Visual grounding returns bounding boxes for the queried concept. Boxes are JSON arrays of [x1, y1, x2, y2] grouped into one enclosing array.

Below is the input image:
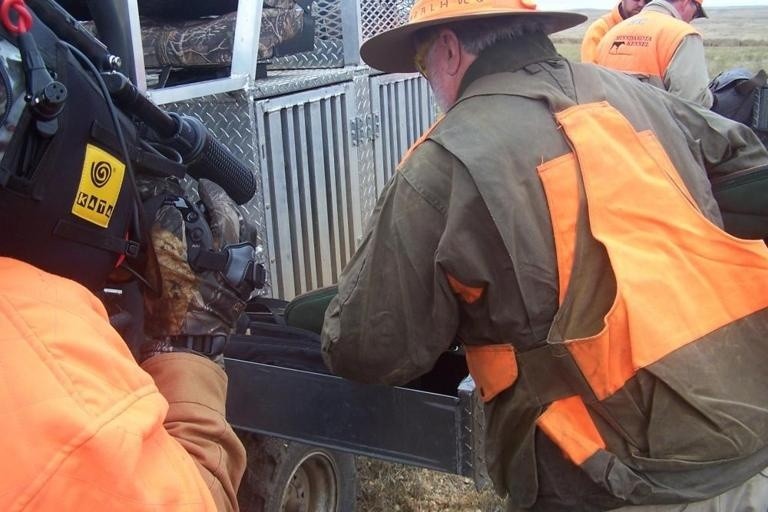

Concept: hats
[[360, 0, 588, 73], [694, 0, 707, 17]]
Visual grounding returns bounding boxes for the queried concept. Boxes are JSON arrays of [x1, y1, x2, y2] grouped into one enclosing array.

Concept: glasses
[[694, 3, 704, 18], [413, 26, 440, 78]]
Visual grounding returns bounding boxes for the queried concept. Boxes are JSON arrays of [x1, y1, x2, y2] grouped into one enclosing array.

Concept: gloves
[[140, 178, 257, 370]]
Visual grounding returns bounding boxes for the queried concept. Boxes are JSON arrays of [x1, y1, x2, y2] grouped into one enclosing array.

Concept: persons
[[575, 0, 654, 68], [313, 0, 768, 509], [604, 0, 722, 113], [1, 172, 262, 512]]
